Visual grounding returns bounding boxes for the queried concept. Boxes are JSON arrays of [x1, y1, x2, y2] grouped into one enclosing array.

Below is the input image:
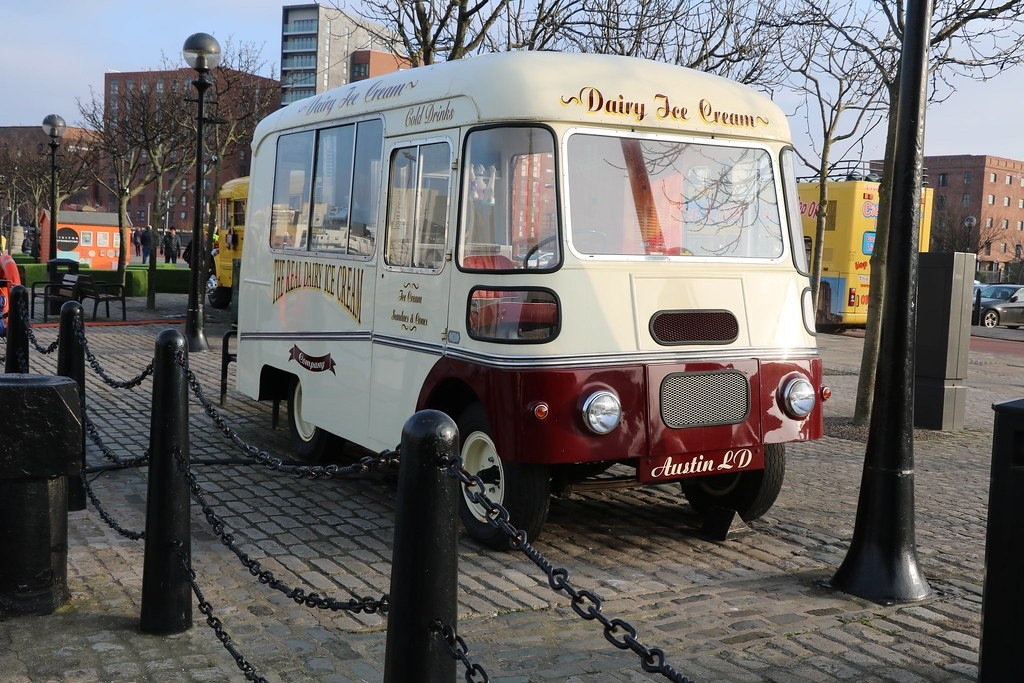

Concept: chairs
[[464, 255, 514, 270]]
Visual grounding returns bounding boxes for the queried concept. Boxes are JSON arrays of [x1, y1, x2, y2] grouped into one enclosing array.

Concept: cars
[[971, 285, 1024, 329]]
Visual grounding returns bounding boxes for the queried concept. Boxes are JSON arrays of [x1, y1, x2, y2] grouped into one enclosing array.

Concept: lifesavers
[[0, 254, 21, 304]]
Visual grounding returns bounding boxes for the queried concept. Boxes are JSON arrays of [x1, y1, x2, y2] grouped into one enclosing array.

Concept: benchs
[[30, 272, 127, 322]]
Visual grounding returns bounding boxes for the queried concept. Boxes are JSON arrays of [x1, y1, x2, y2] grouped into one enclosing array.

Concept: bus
[[795, 160, 935, 334], [203, 171, 326, 309], [236, 50, 832, 554]]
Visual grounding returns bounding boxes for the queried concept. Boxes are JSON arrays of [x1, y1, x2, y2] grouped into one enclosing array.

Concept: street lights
[[43, 114, 66, 260], [183, 33, 222, 352], [965, 216, 976, 253]]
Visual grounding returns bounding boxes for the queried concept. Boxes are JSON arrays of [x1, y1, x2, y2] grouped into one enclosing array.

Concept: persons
[[22, 235, 41, 257], [160, 226, 181, 264], [133, 225, 152, 264], [182, 230, 219, 270]]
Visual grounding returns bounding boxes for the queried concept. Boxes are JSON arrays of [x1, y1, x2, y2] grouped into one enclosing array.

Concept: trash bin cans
[[0, 373, 87, 624], [47, 259, 79, 315]]
